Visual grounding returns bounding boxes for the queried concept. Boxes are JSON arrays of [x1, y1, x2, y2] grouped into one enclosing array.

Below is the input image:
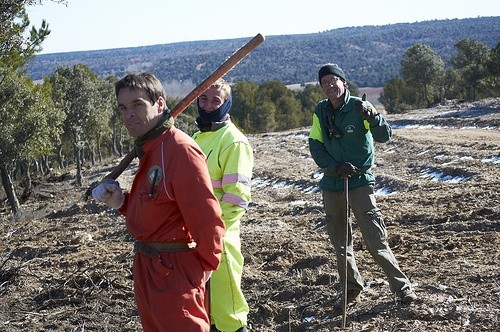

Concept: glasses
[[320, 77, 339, 86]]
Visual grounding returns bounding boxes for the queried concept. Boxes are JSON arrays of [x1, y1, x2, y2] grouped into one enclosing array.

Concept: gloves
[[336, 161, 358, 178], [91, 178, 125, 209], [357, 93, 378, 121]]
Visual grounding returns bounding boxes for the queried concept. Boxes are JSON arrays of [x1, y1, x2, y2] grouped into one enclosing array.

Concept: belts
[[133, 241, 188, 253]]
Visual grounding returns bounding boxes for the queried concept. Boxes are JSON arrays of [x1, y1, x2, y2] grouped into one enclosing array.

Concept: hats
[[319, 63, 346, 88]]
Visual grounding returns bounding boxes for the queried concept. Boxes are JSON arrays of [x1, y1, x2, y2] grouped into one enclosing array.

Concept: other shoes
[[347, 289, 360, 303], [401, 291, 417, 303]]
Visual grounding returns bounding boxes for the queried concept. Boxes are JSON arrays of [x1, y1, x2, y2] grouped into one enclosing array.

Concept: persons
[[191, 77, 254, 332], [92, 71, 225, 332], [309, 63, 417, 307]]
